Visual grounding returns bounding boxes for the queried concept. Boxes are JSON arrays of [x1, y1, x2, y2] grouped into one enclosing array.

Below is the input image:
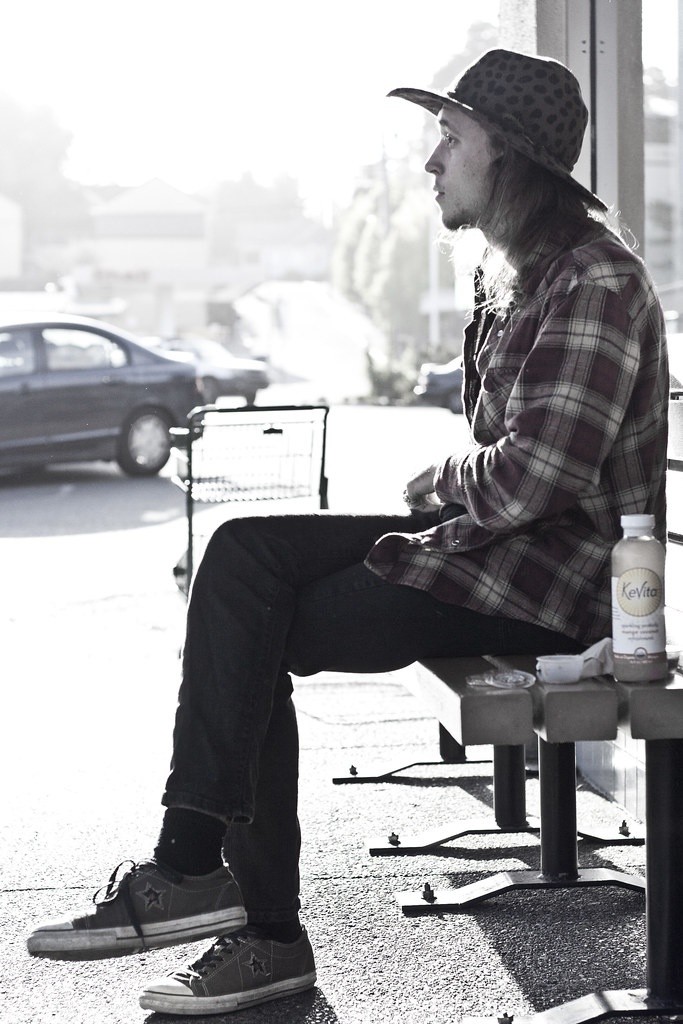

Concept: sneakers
[[140, 926, 316, 1013], [26, 863, 244, 959]]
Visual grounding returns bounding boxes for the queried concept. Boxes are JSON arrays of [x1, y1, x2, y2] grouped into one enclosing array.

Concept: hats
[[386, 49, 611, 214]]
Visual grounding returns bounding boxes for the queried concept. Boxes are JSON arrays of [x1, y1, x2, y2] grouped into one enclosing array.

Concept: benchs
[[407, 652, 683, 1024]]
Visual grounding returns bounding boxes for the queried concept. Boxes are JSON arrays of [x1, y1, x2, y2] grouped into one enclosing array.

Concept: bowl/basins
[[535, 656, 583, 684]]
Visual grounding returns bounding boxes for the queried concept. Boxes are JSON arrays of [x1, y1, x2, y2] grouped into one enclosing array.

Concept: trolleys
[[168, 401, 331, 660]]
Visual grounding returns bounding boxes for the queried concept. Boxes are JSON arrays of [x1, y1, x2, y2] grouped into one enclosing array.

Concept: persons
[[29, 49, 670, 1013]]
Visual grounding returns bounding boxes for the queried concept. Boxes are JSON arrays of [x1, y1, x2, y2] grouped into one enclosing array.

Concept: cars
[[0, 305, 207, 478], [155, 333, 271, 406], [412, 353, 464, 418]]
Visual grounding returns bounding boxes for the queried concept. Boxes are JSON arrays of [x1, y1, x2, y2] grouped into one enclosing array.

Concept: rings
[[403, 490, 418, 503]]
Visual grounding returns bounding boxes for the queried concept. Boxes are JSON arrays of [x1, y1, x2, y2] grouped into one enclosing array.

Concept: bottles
[[609, 514, 671, 683]]
